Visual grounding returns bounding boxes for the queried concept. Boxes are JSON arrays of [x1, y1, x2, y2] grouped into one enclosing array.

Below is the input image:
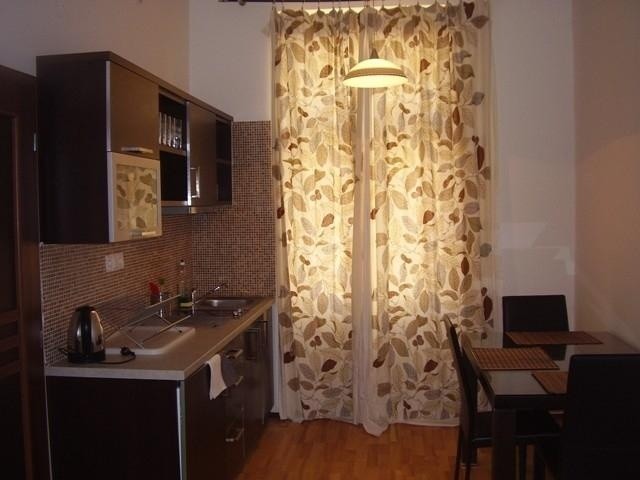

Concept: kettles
[[67, 305, 106, 365]]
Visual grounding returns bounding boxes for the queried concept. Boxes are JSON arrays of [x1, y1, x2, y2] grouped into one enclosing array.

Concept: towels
[[204, 352, 229, 402]]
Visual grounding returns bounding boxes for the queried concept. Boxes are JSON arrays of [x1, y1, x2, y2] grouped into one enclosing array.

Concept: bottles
[[177, 259, 193, 307]]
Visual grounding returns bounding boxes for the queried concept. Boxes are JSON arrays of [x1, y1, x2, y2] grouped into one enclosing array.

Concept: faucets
[[192, 282, 228, 304]]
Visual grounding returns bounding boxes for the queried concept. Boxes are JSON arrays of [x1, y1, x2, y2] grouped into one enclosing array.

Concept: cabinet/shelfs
[[186, 351, 246, 480], [159, 92, 192, 206], [239, 307, 274, 457], [106, 63, 163, 242], [191, 102, 234, 207]]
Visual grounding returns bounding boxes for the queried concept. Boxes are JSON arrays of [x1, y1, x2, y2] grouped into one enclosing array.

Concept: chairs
[[440, 290, 639, 479]]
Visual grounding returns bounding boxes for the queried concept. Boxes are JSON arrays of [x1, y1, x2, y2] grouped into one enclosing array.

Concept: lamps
[[340, 4, 409, 88]]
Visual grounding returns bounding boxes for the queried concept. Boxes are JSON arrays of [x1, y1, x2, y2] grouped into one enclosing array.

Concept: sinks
[[192, 297, 256, 311]]
